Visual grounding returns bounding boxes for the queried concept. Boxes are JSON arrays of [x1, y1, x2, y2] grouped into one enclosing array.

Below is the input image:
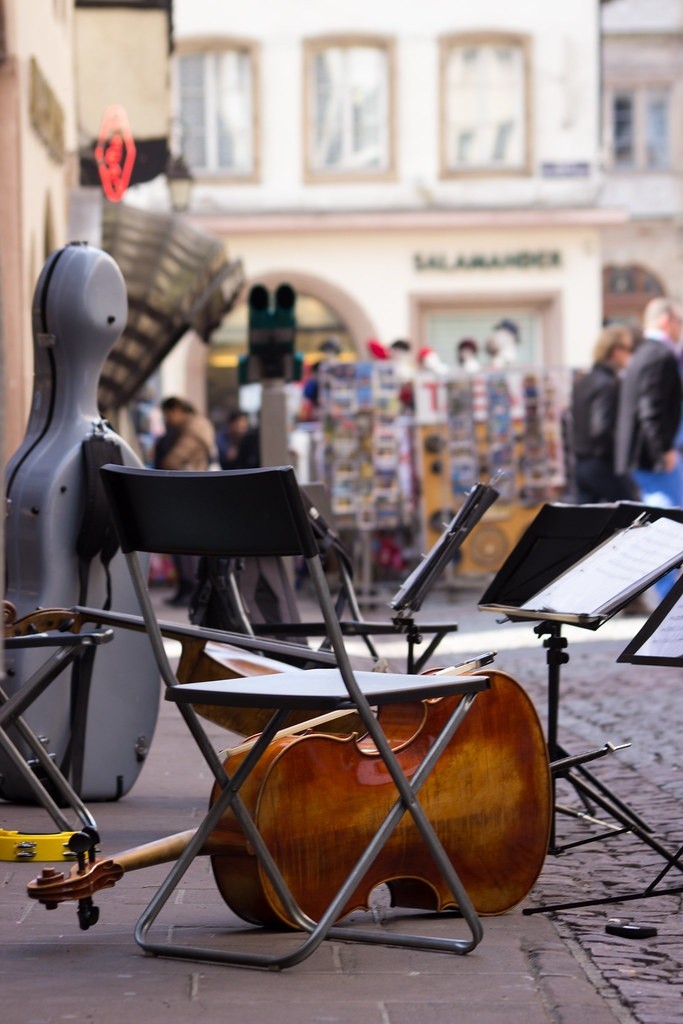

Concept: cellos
[[0, 598, 389, 739], [28, 670, 555, 932]]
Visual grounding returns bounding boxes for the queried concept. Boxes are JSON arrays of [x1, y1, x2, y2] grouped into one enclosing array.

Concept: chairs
[[100, 461, 491, 977]]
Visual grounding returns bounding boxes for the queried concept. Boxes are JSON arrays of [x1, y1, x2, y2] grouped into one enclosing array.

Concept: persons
[[559, 297, 683, 610], [150, 398, 260, 606]]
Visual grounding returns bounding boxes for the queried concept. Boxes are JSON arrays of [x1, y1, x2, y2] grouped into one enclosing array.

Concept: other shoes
[[165, 591, 197, 607]]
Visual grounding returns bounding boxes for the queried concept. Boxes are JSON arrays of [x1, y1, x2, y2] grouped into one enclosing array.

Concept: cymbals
[[0, 827, 101, 862]]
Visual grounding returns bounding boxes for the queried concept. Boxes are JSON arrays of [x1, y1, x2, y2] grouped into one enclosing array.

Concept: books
[[477, 500, 683, 622]]
